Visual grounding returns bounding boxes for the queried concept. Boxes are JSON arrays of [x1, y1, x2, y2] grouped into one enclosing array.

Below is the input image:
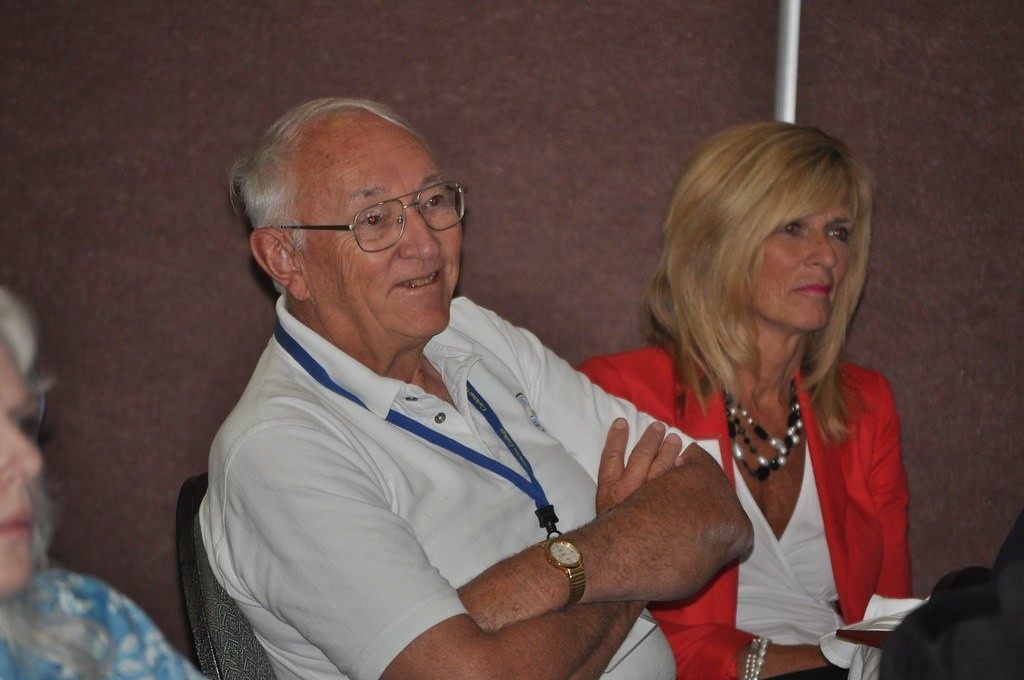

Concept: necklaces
[[723, 380, 804, 483]]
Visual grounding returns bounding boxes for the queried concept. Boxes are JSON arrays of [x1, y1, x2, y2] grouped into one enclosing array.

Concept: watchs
[[537, 537, 584, 608]]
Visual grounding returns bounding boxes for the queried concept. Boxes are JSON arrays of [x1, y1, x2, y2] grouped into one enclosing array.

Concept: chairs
[[174, 470, 276, 680]]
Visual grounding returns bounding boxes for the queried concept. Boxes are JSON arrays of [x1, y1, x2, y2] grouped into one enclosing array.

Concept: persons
[[197, 99, 759, 680], [0, 290, 212, 679], [577, 119, 912, 680]]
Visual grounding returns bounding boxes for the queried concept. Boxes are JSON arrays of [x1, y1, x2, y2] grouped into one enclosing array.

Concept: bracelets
[[744, 635, 770, 680]]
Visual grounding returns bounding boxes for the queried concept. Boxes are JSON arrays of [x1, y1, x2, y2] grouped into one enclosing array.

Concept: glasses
[[278, 180, 467, 253]]
